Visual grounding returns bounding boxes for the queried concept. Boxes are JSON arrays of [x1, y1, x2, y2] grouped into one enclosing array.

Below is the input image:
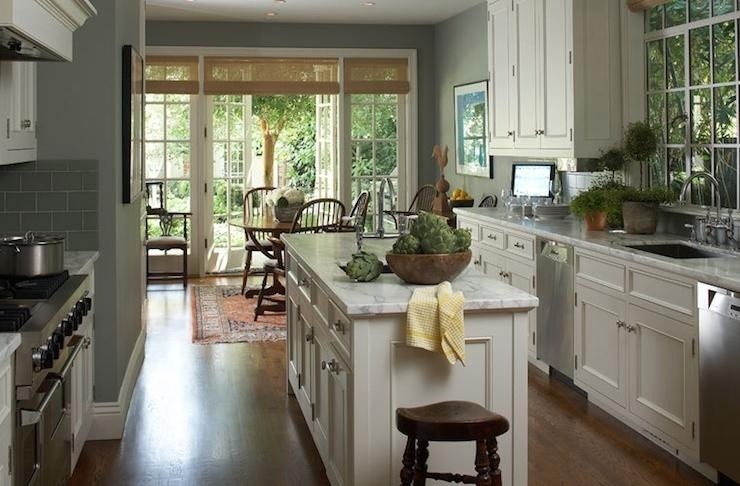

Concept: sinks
[[621, 243, 728, 258]]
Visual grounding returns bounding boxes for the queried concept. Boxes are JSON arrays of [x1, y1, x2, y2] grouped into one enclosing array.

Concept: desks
[[227, 213, 339, 315]]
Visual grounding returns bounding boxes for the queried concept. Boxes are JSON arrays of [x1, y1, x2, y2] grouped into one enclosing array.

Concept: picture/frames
[[123, 44, 145, 204], [453, 79, 493, 178]]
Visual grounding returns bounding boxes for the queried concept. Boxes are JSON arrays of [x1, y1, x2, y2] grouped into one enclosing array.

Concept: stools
[[396, 400, 509, 486]]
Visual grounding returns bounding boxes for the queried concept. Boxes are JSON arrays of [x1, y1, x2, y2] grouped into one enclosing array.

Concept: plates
[[534, 204, 572, 221]]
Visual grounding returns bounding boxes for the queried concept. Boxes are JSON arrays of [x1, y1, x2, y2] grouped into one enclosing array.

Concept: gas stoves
[[0, 271, 91, 373]]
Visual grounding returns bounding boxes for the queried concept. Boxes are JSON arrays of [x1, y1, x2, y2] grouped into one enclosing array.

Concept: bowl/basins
[[448, 199, 474, 212], [274, 203, 302, 224], [384, 252, 473, 286]]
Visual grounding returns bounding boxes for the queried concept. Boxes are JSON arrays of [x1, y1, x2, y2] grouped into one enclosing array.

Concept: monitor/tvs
[[511, 163, 555, 197]]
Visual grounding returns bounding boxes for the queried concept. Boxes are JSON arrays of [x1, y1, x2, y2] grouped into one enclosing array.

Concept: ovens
[[11, 332, 86, 485]]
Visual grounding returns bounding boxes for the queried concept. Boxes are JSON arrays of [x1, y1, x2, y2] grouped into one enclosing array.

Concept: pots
[[1, 233, 67, 279]]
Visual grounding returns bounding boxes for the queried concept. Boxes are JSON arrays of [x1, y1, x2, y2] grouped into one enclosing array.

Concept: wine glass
[[516, 189, 530, 220], [500, 189, 513, 219], [549, 179, 561, 204]]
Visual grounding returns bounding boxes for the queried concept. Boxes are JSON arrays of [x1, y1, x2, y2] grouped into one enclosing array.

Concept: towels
[[406, 281, 466, 367]]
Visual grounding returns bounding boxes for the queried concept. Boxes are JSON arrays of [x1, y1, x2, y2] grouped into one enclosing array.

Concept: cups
[[531, 198, 551, 221]]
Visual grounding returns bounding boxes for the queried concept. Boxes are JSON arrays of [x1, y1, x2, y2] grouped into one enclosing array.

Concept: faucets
[[679, 173, 721, 225]]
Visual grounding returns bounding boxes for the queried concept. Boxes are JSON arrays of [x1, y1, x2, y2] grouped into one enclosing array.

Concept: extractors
[[0, 0, 99, 64]]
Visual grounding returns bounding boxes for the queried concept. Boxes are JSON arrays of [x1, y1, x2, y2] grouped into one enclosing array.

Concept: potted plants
[[573, 120, 679, 234]]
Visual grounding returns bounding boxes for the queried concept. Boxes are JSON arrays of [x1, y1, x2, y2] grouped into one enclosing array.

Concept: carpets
[[191, 285, 287, 346]]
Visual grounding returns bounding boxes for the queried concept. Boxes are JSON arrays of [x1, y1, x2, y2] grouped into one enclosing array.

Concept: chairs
[[146, 212, 192, 289], [322, 190, 370, 232], [240, 186, 285, 294], [383, 185, 437, 230], [454, 195, 497, 231], [254, 197, 345, 322]]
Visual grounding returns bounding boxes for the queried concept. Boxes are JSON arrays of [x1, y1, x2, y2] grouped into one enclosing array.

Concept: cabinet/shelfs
[[456, 215, 538, 357], [0, 61, 38, 168], [486, 0, 621, 160], [573, 246, 699, 450], [279, 232, 538, 486], [0, 355, 15, 486], [70, 263, 95, 476]]
